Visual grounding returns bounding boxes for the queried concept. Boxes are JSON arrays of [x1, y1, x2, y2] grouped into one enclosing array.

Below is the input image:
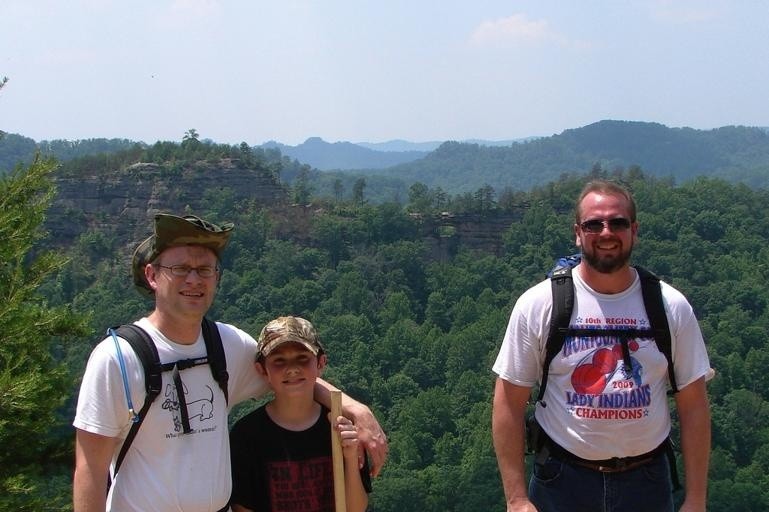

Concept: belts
[[571, 457, 653, 474]]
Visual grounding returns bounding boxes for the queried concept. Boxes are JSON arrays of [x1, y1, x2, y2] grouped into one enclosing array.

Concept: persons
[[490, 178, 715, 512], [226, 314, 373, 511], [70, 242, 389, 512]]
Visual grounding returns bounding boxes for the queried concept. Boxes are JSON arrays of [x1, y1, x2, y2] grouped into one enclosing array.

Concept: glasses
[[156, 262, 220, 278], [579, 217, 634, 234]]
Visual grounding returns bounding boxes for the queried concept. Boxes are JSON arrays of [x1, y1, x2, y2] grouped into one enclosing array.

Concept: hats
[[130, 211, 235, 303], [255, 315, 326, 357]]
